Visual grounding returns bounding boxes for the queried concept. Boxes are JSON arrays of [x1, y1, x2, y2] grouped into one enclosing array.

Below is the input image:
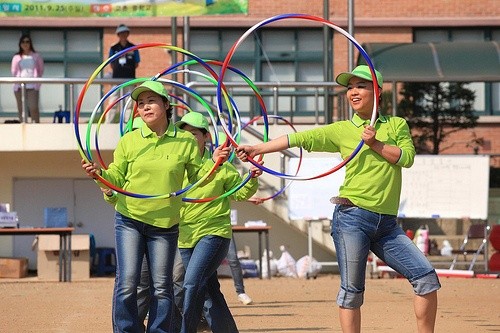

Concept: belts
[[330, 196, 354, 206]]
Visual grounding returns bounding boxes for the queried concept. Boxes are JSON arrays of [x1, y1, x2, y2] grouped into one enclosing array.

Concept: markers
[[461, 216, 470, 218]]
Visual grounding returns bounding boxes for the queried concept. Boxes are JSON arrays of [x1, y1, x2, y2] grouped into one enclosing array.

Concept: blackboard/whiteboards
[[287, 153, 489, 223]]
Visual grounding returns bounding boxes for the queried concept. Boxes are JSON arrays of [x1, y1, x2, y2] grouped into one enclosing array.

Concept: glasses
[[21, 40, 30, 44]]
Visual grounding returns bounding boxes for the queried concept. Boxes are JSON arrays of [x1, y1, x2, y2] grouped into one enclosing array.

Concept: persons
[[101, 25, 140, 124], [11, 34, 43, 124], [237, 65, 441, 333], [81, 79, 265, 333]]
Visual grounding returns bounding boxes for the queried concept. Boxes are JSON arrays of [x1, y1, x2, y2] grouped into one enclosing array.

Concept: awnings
[[0, 0, 248, 127], [351, 42, 500, 118]]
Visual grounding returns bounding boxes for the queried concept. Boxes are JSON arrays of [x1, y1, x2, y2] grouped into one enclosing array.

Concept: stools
[[53, 110, 69, 124], [91, 247, 117, 278]]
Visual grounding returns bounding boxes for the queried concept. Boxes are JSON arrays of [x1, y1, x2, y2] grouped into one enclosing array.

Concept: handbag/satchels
[[414, 225, 429, 256]]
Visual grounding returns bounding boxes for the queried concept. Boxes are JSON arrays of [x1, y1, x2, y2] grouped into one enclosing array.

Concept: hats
[[131, 80, 167, 100], [175, 112, 208, 132], [336, 65, 383, 88], [115, 26, 130, 34]]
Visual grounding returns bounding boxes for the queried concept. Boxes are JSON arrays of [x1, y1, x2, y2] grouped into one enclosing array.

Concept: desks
[[231, 224, 272, 280], [0, 227, 76, 283]]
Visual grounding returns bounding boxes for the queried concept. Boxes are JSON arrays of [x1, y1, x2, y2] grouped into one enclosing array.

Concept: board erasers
[[432, 214, 439, 219], [398, 213, 404, 218]]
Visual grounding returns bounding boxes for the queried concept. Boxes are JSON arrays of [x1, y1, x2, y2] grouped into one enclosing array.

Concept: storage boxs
[[31, 233, 90, 279], [0, 257, 28, 278], [43, 207, 67, 228]]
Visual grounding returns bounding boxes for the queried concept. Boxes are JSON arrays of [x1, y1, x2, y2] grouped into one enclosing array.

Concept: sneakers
[[239, 293, 251, 305]]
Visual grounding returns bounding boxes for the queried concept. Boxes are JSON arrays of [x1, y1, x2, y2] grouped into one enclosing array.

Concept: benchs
[[427, 235, 485, 262]]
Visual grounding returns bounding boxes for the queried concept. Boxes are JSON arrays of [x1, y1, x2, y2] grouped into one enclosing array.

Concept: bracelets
[[93, 169, 102, 180], [248, 169, 256, 179], [212, 158, 223, 166], [106, 193, 114, 197]]
